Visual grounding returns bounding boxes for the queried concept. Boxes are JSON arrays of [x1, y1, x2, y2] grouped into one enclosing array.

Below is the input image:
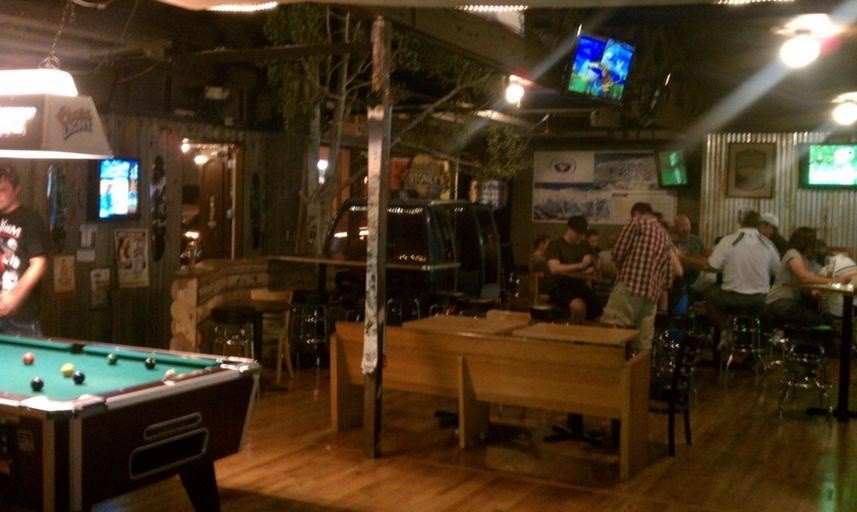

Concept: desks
[[1, 331, 266, 512]]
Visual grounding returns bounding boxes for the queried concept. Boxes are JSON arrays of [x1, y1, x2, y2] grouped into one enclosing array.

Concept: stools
[[206, 252, 708, 482]]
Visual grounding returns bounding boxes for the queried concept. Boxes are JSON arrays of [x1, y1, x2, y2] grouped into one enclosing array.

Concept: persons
[[707, 210, 856, 353], [530, 215, 618, 324], [652, 209, 709, 312], [602, 201, 675, 359], [1, 163, 50, 341]]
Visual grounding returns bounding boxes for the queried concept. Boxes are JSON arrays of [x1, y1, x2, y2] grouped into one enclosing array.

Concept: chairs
[[705, 251, 856, 422]]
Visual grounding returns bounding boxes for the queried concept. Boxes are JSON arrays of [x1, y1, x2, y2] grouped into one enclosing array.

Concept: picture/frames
[[727, 141, 776, 198]]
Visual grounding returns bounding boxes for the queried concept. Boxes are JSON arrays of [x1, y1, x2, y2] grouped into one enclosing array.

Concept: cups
[[590, 255, 618, 278], [185, 239, 203, 271], [824, 257, 836, 279]]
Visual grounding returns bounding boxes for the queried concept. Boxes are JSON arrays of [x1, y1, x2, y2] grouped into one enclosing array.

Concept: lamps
[[0, 68, 115, 161]]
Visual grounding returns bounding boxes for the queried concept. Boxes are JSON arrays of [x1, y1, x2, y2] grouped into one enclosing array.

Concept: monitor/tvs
[[95, 157, 141, 220], [655, 148, 690, 188], [807, 142, 857, 189], [560, 26, 635, 107]]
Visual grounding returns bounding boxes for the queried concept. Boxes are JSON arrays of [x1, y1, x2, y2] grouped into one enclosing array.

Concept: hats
[[760, 213, 780, 229], [567, 215, 591, 235]]
